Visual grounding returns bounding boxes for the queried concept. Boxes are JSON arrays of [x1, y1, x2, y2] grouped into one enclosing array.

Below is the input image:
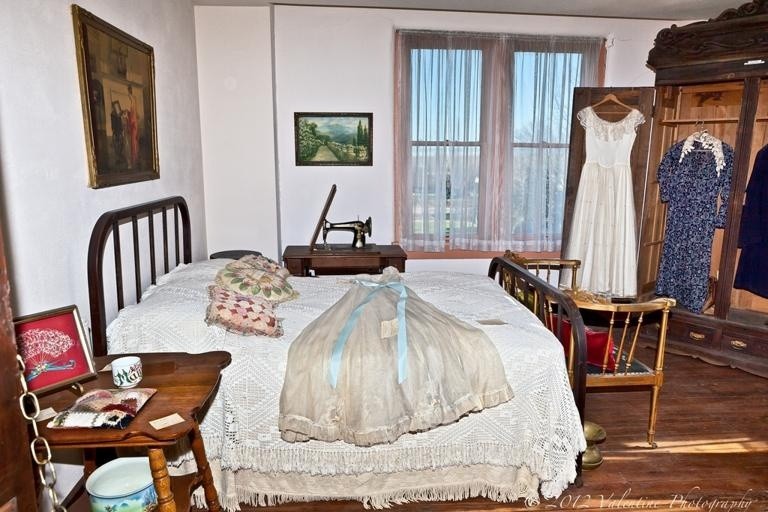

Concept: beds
[[87, 196, 588, 505]]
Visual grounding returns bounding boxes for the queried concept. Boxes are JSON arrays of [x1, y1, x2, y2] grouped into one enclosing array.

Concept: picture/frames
[[293, 112, 373, 166], [12, 303, 99, 400], [69, 2, 162, 189]]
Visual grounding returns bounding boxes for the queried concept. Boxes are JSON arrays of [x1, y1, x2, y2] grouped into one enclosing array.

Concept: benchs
[[500, 249, 678, 447]]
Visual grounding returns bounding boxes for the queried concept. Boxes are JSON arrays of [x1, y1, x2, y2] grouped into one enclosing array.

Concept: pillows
[[204, 255, 300, 338]]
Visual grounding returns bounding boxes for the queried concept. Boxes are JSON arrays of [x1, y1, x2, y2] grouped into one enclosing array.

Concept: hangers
[[687, 119, 712, 152], [591, 87, 633, 115]]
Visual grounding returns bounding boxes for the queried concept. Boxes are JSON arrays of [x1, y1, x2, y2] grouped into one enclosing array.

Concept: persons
[[110, 100, 126, 166], [127, 86, 140, 169]]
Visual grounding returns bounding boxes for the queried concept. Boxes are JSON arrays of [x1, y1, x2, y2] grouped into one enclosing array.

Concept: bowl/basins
[[85, 456, 159, 512]]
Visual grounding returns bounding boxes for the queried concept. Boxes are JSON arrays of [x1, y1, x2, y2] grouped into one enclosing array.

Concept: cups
[[111, 356, 143, 389]]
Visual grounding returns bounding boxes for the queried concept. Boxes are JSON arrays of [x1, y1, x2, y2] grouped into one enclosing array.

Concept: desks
[[26, 351, 232, 512], [282, 245, 407, 277]]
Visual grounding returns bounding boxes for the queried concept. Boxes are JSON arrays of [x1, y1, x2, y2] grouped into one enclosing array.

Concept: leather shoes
[[581, 441, 603, 470], [583, 420, 606, 444]]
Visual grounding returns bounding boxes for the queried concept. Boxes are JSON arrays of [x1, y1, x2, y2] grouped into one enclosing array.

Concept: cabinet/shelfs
[[558, 0, 768, 379]]
